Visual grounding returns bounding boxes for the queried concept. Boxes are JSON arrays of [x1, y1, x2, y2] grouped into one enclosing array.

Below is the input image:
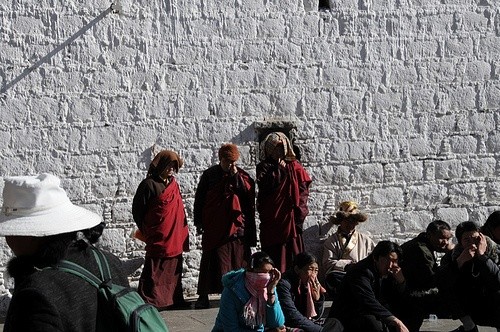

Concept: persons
[[211, 200, 500, 332], [256, 132, 312, 276], [0, 173, 130, 332], [132, 150, 190, 309], [193, 143, 256, 308]]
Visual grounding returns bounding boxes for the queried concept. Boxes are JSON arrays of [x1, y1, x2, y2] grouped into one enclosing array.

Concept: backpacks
[[48, 244, 168, 332]]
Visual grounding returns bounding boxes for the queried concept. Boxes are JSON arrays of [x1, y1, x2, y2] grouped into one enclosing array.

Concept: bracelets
[[269, 292, 276, 296]]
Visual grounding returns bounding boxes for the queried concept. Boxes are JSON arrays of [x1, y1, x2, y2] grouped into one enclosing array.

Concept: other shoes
[[196, 298, 210, 309]]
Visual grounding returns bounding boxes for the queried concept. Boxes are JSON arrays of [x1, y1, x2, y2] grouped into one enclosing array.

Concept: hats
[[0, 173, 101, 236], [329, 201, 367, 227]]
